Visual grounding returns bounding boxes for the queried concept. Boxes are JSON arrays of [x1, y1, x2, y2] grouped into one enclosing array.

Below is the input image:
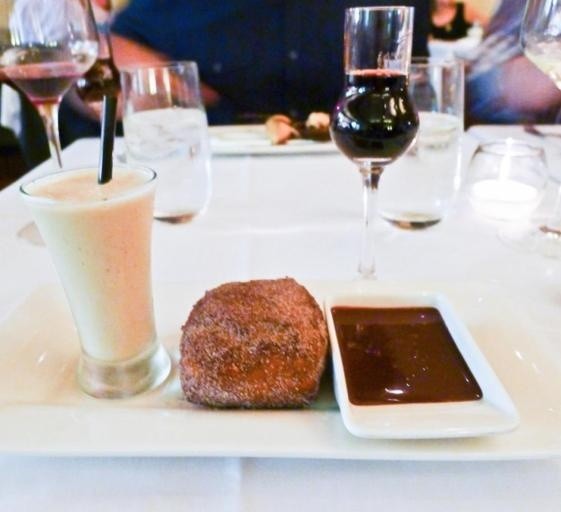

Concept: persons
[[0, 0, 560, 149]]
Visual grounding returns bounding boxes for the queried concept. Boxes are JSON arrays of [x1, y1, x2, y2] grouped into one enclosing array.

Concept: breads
[[177, 278, 329, 409]]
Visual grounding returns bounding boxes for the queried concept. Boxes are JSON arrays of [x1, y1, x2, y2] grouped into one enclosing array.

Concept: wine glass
[[0, 0, 99, 195], [329, 70, 422, 240]]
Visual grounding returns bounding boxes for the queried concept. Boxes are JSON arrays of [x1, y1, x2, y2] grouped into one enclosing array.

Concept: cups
[[374, 55, 464, 231], [118, 58, 214, 226], [465, 140, 553, 234], [18, 163, 174, 400], [342, 4, 415, 231]]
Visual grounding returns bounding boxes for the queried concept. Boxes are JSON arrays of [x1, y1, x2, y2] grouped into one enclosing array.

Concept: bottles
[[74, 1, 121, 124]]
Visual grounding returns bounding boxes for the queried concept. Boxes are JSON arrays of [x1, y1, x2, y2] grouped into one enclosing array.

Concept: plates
[[321, 280, 525, 442]]
